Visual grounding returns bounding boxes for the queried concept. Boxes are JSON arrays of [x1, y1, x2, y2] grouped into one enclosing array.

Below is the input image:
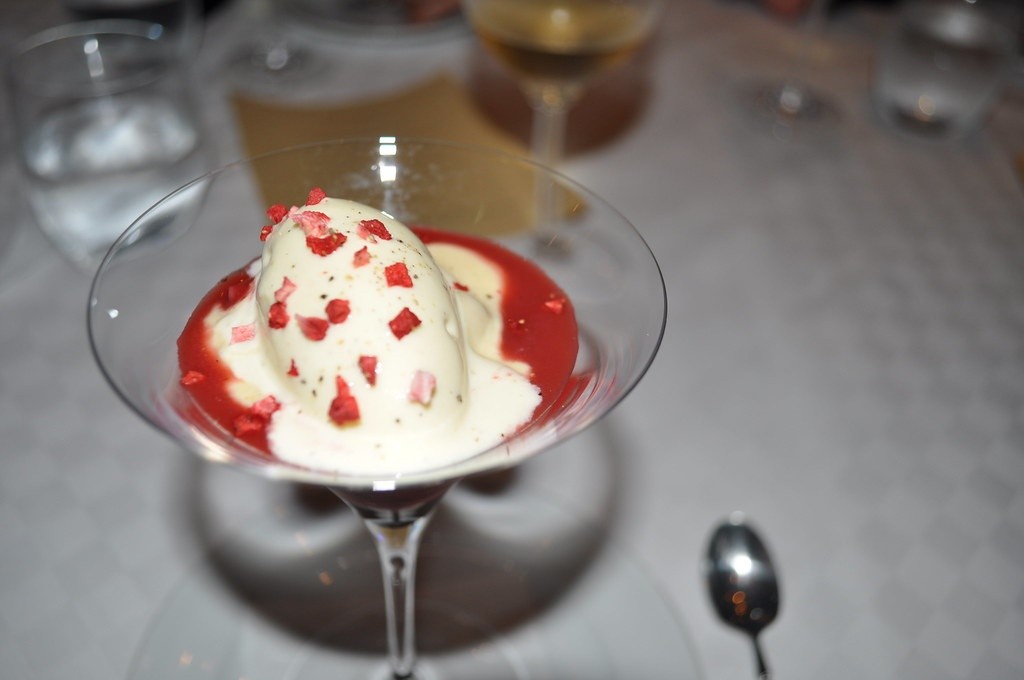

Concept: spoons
[[705, 522, 780, 680]]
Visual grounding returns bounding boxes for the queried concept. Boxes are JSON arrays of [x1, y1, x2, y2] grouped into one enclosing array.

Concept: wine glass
[[86, 137, 670, 680]]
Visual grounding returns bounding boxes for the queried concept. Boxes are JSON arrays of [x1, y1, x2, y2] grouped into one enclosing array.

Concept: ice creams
[[171, 184, 579, 473]]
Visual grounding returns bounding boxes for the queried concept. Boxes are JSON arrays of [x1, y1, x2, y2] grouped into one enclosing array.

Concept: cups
[[0, 19, 213, 279], [869, 0, 1024, 146], [463, 0, 666, 152]]
